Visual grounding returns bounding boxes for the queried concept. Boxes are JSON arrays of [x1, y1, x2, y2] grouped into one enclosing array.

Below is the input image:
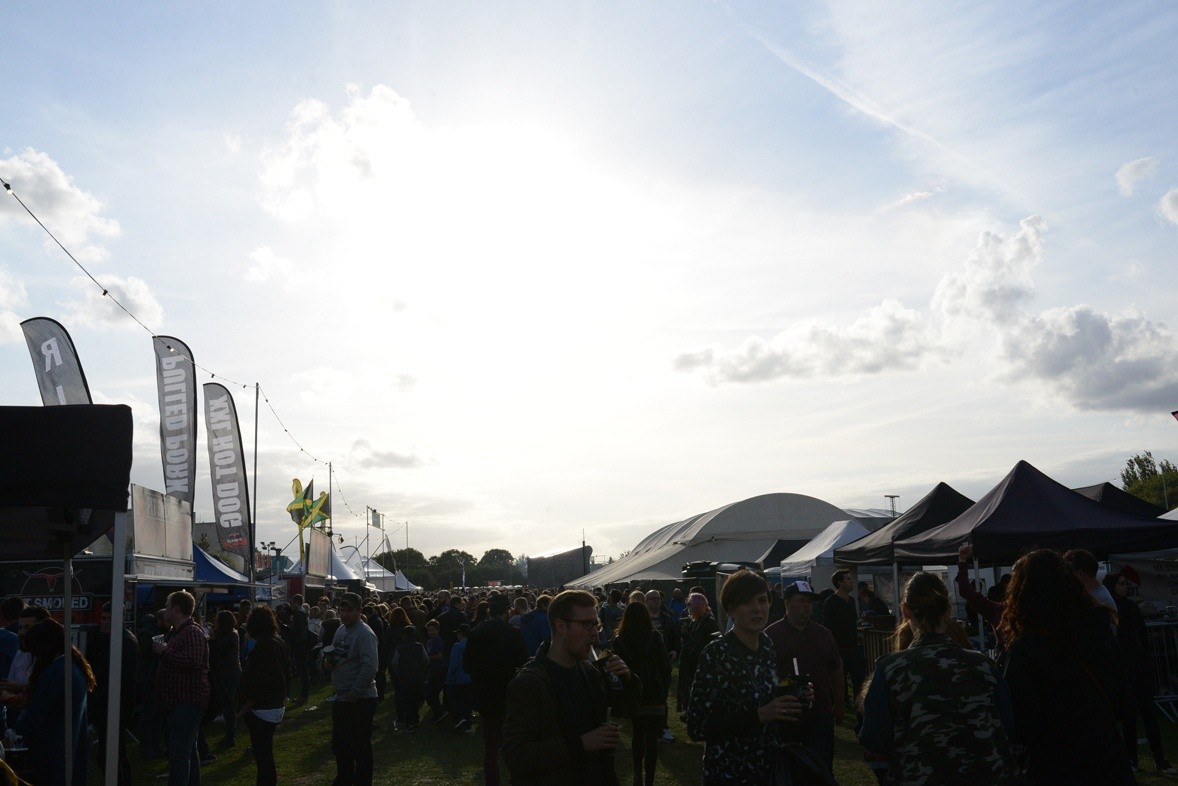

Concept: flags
[[287, 479, 331, 528], [152, 335, 196, 504], [203, 383, 251, 563], [19, 317, 92, 406]]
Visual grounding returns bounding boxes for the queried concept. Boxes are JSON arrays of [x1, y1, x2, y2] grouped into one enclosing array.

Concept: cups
[[152, 634, 165, 646], [601, 723, 618, 754], [789, 672, 810, 703], [773, 681, 796, 697], [594, 657, 611, 677], [323, 645, 336, 664]]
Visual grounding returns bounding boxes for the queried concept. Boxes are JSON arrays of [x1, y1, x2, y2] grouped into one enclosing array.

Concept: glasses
[[648, 597, 662, 602], [562, 619, 602, 631]]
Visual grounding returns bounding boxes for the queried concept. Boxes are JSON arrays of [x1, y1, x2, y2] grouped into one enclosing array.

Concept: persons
[[462, 594, 528, 786], [132, 599, 253, 779], [859, 542, 1178, 786], [593, 586, 716, 743], [687, 569, 865, 786], [272, 594, 341, 704], [87, 599, 139, 786], [151, 591, 210, 786], [0, 596, 96, 786], [236, 605, 290, 786], [612, 601, 673, 786], [323, 592, 379, 786], [501, 589, 646, 786], [361, 586, 554, 735]]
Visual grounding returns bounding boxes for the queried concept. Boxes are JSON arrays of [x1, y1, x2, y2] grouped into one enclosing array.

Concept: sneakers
[[659, 730, 675, 743]]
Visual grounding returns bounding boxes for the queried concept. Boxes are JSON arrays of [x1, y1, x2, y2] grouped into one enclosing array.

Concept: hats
[[338, 592, 363, 611], [785, 581, 813, 599], [452, 624, 472, 633]]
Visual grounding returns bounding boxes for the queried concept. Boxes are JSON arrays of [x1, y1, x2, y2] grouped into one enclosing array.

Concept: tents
[[566, 461, 1178, 652], [136, 543, 271, 614]]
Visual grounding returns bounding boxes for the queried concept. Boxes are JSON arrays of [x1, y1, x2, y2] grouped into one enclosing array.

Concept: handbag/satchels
[[772, 743, 836, 786]]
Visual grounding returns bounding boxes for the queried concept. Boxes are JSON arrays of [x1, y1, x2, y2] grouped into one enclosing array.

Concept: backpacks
[[617, 630, 657, 687]]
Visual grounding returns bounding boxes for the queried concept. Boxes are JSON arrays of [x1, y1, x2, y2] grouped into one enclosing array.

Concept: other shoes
[[199, 755, 217, 763], [433, 712, 449, 723], [452, 718, 468, 729], [1158, 769, 1178, 779], [1131, 766, 1144, 773], [465, 725, 472, 732], [218, 740, 234, 749]]
